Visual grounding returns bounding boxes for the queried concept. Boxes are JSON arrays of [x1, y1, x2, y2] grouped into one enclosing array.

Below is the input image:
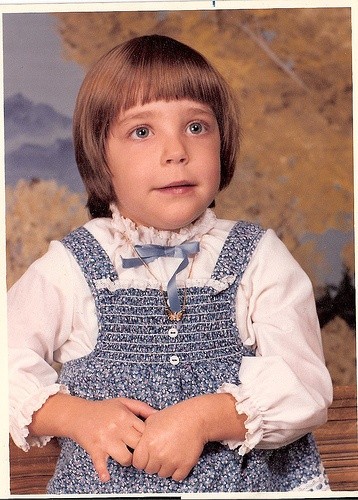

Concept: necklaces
[[114, 230, 202, 321]]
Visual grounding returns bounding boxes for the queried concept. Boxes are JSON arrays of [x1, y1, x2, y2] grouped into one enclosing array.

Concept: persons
[[6, 35, 333, 493]]
